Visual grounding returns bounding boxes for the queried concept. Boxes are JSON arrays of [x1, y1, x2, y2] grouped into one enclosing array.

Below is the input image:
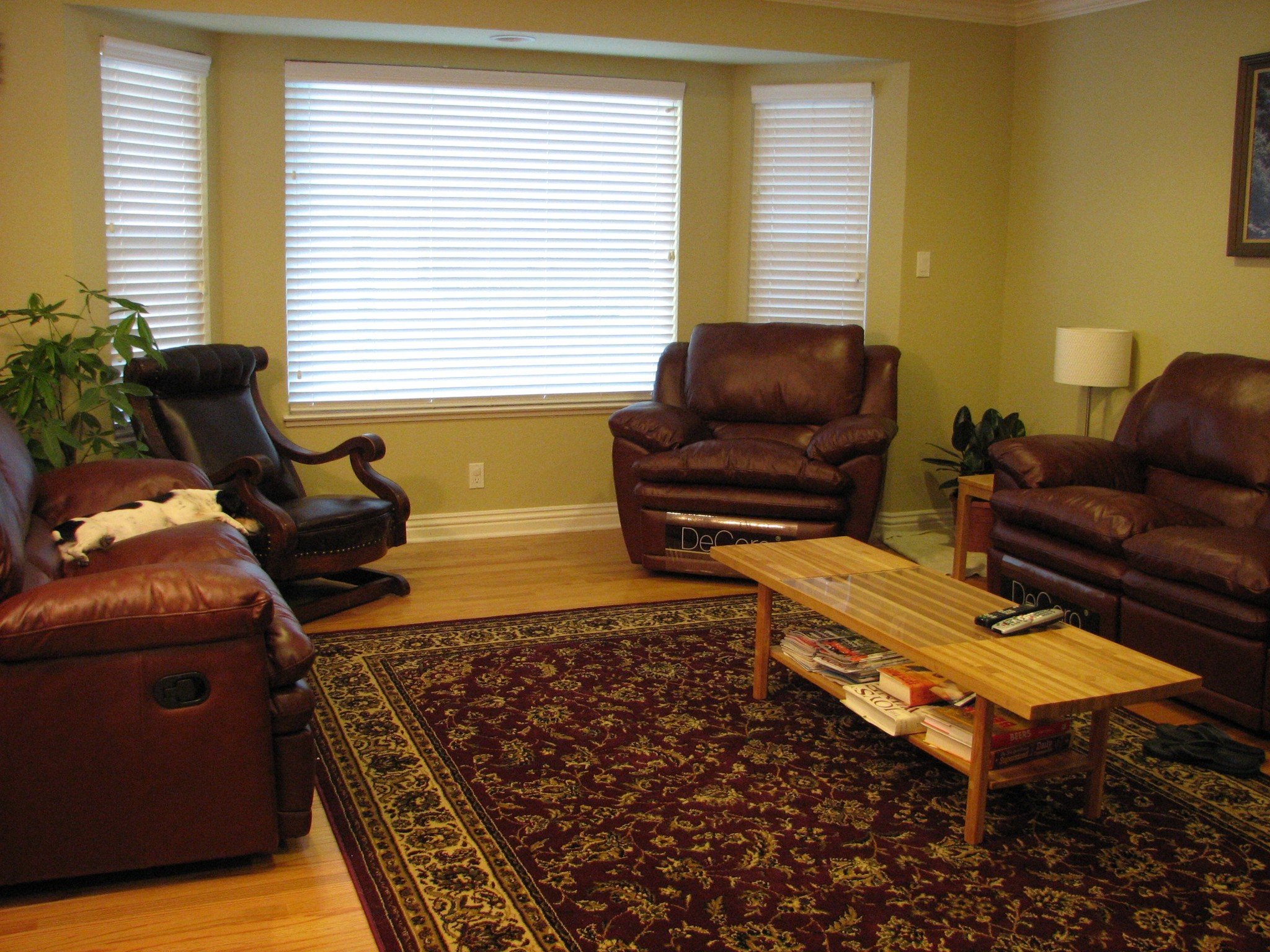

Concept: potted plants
[[926, 407, 1028, 553]]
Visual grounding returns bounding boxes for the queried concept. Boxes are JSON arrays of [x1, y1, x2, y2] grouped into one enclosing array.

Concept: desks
[[951, 471, 993, 584], [709, 537, 1202, 847]]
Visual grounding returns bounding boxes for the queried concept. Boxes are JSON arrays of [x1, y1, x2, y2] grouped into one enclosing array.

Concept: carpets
[[303, 590, 1270, 952]]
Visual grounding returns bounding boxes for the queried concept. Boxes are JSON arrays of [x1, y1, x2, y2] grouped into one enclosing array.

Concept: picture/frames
[[1221, 51, 1270, 258]]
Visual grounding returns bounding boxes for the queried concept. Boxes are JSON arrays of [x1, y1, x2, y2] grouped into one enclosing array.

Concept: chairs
[[123, 344, 410, 625]]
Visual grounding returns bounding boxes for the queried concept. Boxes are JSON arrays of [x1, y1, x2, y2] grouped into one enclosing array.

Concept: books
[[780, 625, 1075, 770]]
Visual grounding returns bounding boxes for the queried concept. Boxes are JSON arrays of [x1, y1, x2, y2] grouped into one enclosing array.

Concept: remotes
[[975, 605, 1042, 627], [991, 608, 1064, 635]]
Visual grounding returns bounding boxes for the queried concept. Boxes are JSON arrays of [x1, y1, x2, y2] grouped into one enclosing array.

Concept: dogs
[[51, 488, 265, 568]]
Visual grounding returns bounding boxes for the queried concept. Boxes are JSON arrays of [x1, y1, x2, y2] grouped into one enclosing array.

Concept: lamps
[[1051, 327, 1133, 437]]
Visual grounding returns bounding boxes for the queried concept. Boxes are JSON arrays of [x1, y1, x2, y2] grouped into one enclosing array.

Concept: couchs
[[0, 402, 319, 887], [981, 352, 1270, 733], [608, 323, 903, 579]]
